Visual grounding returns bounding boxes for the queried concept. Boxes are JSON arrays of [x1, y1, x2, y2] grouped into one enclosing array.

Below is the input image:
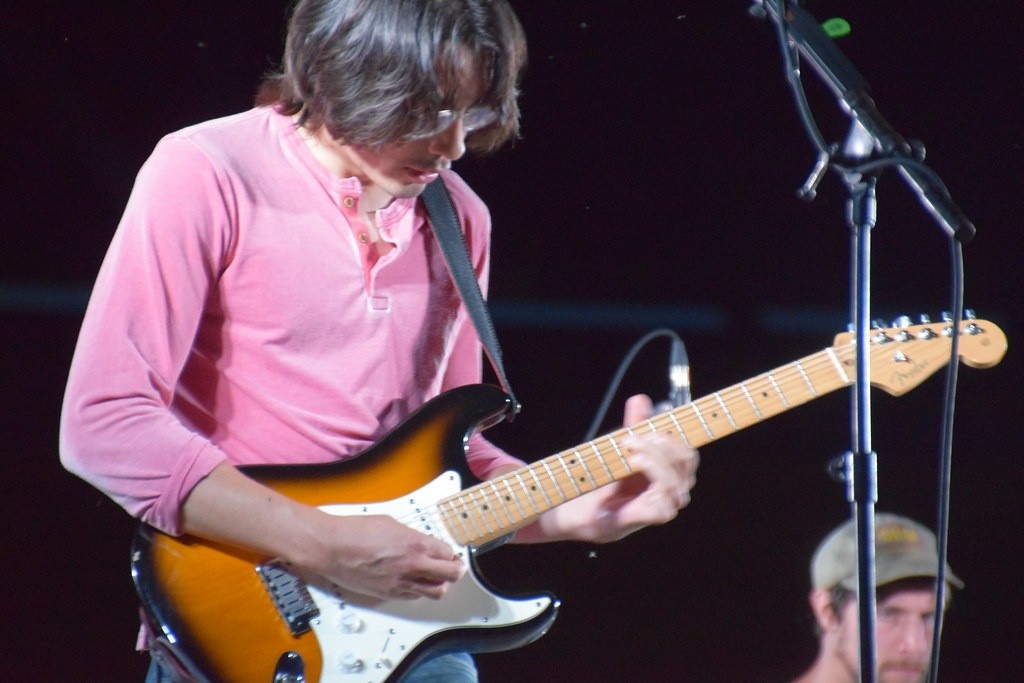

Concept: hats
[[810, 513, 967, 592]]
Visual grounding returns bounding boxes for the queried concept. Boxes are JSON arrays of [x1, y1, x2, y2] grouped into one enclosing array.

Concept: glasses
[[400, 105, 502, 139]]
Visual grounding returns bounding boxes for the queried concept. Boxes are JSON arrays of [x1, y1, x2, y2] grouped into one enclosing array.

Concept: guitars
[[129, 306, 1010, 683]]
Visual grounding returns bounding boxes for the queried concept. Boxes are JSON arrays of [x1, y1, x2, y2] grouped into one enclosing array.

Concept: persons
[[791, 513, 965, 683], [59, 2, 700, 681]]
